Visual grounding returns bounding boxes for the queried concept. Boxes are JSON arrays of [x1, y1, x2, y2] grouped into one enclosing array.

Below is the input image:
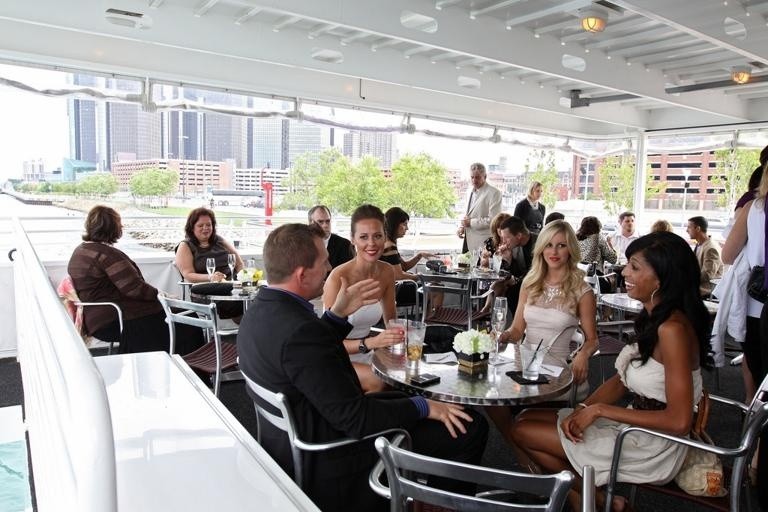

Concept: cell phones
[[409, 373, 440, 388]]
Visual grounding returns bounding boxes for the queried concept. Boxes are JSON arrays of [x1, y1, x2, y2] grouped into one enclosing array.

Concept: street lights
[[181, 135, 190, 196]]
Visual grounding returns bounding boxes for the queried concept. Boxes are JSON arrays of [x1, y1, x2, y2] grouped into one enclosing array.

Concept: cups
[[388, 319, 410, 348], [518, 343, 545, 379], [489, 257, 493, 269], [402, 322, 426, 370], [234, 240, 240, 249], [493, 252, 502, 272]]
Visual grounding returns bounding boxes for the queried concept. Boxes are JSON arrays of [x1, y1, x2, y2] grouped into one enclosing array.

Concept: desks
[[366, 329, 578, 408], [407, 259, 513, 285], [596, 289, 721, 316], [186, 276, 265, 303]]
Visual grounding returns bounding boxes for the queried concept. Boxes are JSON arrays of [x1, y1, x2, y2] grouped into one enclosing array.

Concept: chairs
[[369, 434, 595, 512], [61, 241, 632, 384], [234, 355, 415, 490], [154, 292, 240, 399], [601, 369, 768, 512]]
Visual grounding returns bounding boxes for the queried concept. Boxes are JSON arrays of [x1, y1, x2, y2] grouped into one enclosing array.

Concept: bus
[[207, 187, 265, 208]]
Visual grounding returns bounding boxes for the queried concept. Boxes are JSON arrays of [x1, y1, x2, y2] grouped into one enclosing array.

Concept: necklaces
[[543, 281, 562, 304]]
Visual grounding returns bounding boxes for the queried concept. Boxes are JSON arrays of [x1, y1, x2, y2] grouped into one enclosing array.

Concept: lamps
[[730, 66, 752, 84], [577, 11, 609, 33]]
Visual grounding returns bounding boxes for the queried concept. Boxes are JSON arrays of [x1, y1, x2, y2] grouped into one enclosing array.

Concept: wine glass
[[207, 258, 216, 282], [245, 261, 256, 294], [227, 254, 237, 289], [489, 297, 507, 363]]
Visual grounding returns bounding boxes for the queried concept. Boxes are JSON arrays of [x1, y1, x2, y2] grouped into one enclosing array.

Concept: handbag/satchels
[[673, 388, 728, 497], [746, 264, 768, 305]]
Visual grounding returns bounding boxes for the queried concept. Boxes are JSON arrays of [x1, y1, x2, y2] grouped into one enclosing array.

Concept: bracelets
[[578, 402, 587, 407]]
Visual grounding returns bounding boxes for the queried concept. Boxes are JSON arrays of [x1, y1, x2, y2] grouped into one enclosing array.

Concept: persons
[[174, 208, 244, 320], [307, 206, 357, 281], [721, 145, 768, 467], [512, 231, 717, 511], [321, 204, 399, 395], [67, 205, 212, 388], [457, 163, 502, 254], [686, 217, 723, 280], [651, 220, 672, 232], [378, 207, 444, 307], [237, 224, 489, 512], [490, 219, 599, 474], [513, 181, 546, 231], [481, 212, 646, 319]]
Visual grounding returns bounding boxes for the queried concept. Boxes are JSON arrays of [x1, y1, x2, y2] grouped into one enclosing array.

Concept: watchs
[[359, 339, 371, 354]]
